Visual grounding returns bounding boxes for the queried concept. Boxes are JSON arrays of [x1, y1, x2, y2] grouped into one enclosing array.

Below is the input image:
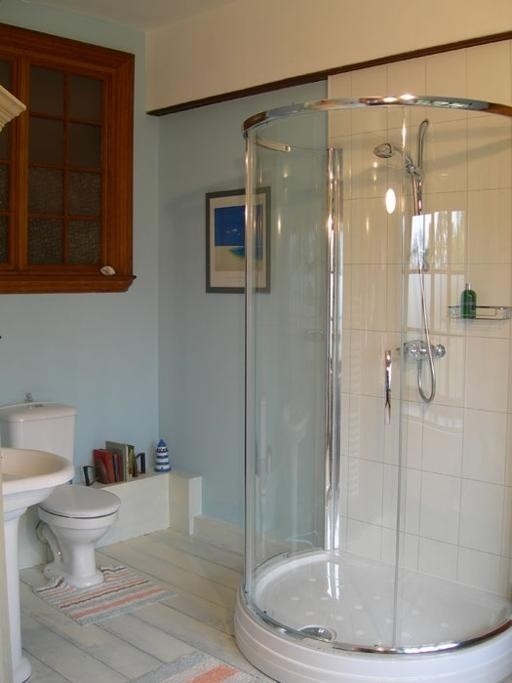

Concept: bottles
[[154, 439, 170, 471], [460, 283, 477, 319]]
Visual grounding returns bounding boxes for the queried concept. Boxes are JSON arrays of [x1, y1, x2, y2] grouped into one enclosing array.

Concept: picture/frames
[[205, 185, 271, 293]]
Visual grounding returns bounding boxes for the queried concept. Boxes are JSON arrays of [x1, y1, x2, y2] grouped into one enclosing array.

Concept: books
[[93, 441, 135, 484]]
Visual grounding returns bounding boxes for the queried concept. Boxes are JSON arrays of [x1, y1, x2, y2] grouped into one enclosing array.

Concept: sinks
[[0, 447, 75, 514]]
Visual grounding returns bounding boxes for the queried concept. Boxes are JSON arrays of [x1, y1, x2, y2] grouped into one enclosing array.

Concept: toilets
[[0, 402, 121, 590]]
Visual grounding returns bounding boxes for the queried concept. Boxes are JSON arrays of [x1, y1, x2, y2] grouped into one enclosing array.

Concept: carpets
[[127, 648, 267, 683], [32, 563, 177, 626]]
